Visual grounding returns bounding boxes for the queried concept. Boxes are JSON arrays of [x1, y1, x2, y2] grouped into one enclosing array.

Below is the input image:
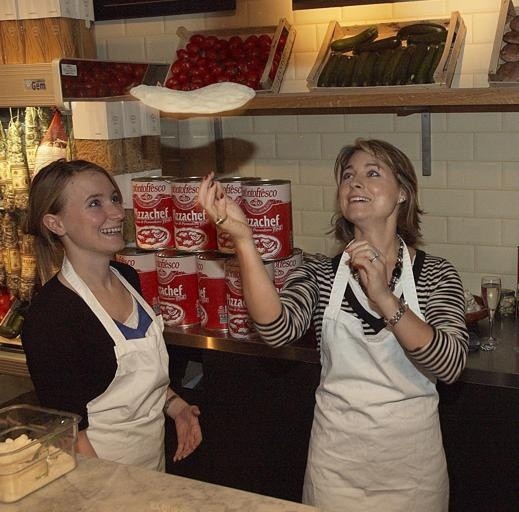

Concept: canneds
[[130, 177, 171, 250], [238, 178, 293, 261], [114, 249, 160, 326], [211, 175, 257, 254], [175, 177, 215, 255], [220, 257, 274, 340], [196, 253, 228, 329], [270, 246, 302, 295], [150, 249, 199, 329]]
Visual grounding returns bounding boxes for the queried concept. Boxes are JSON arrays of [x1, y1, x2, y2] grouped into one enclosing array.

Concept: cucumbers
[[319, 23, 458, 86]]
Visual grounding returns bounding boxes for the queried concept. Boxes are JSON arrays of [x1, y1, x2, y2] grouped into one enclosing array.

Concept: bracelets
[[382, 297, 410, 331], [162, 394, 181, 416]]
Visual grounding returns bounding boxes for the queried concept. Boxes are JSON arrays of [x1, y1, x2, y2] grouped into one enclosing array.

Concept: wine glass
[[481, 275, 503, 346]]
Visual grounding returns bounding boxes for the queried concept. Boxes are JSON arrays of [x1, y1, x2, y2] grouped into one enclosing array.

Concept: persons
[[17, 159, 207, 481], [198, 138, 471, 512]]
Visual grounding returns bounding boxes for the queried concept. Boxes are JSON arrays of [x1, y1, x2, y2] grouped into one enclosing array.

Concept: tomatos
[[64, 59, 148, 97], [163, 33, 284, 91]]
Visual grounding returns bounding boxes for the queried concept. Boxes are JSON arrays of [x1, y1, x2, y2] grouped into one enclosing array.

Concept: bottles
[[499, 288, 516, 320]]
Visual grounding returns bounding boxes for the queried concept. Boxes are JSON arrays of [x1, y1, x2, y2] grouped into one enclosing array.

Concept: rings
[[215, 216, 230, 226], [369, 255, 379, 262]]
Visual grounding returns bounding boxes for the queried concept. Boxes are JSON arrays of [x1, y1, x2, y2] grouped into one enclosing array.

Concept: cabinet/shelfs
[[1, 54, 175, 408]]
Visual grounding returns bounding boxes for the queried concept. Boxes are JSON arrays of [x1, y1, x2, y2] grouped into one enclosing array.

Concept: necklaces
[[348, 232, 404, 292]]
